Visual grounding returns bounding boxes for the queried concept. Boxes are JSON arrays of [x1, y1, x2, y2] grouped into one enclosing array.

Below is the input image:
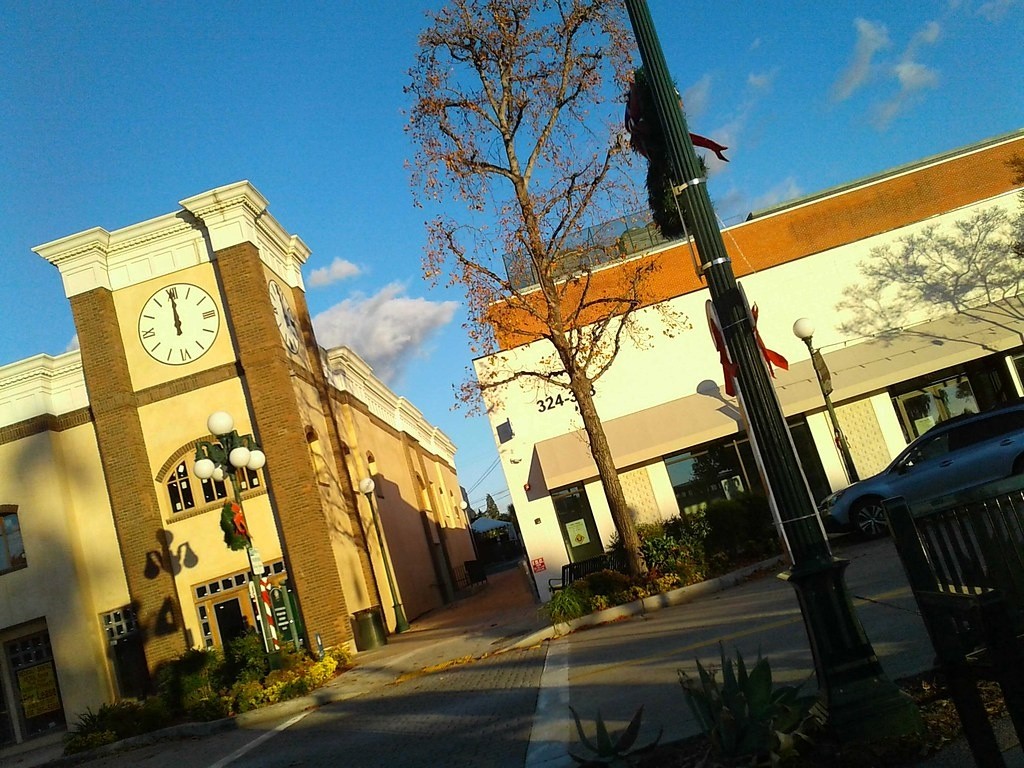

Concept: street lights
[[193, 411, 283, 670], [359, 478, 411, 634], [460, 501, 489, 584], [792, 317, 860, 484]]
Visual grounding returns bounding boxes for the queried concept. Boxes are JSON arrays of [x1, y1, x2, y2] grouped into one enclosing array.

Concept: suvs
[[818, 398, 1024, 538]]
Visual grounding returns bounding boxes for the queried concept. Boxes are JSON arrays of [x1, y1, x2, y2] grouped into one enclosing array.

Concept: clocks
[[137, 283, 220, 365]]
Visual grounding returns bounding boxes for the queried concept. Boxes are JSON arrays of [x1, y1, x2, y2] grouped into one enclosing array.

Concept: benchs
[[548, 552, 613, 597], [882, 469, 1024, 768]]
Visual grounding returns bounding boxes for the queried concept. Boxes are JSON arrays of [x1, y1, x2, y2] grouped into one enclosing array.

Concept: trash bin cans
[[351, 604, 388, 652]]
